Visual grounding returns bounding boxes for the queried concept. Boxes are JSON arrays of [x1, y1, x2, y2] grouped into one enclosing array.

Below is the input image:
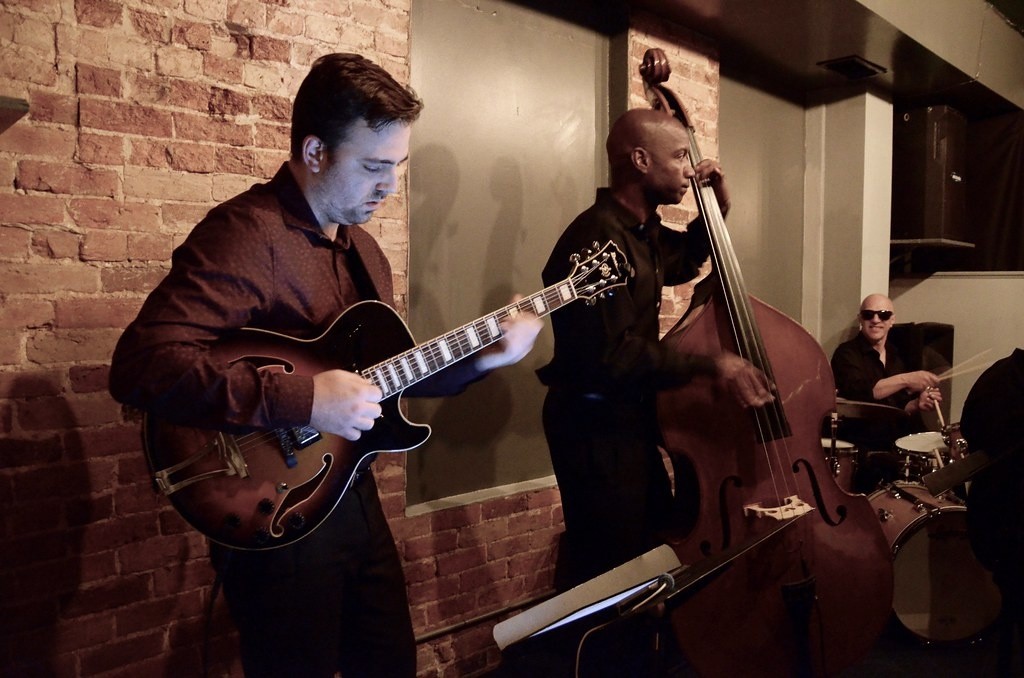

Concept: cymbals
[[827, 396, 911, 420]]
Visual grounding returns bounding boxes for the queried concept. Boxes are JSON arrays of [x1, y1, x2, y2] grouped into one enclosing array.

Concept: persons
[[535, 107, 776, 678], [108, 50, 545, 678], [829, 292, 943, 498]]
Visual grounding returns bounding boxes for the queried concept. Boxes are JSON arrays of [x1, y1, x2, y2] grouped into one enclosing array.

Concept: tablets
[[500, 559, 691, 652]]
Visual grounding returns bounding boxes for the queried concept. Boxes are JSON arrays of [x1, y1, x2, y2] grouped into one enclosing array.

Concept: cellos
[[638, 47, 894, 678]]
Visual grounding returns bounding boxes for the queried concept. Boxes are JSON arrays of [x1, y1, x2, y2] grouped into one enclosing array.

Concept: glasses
[[860, 310, 895, 321]]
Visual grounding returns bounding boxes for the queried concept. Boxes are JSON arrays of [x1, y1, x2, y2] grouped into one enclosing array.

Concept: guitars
[[143, 241, 635, 552]]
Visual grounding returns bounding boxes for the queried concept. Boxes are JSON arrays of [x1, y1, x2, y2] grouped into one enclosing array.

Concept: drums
[[864, 480, 1002, 647], [941, 422, 970, 464], [894, 431, 949, 479], [821, 438, 858, 491]]
